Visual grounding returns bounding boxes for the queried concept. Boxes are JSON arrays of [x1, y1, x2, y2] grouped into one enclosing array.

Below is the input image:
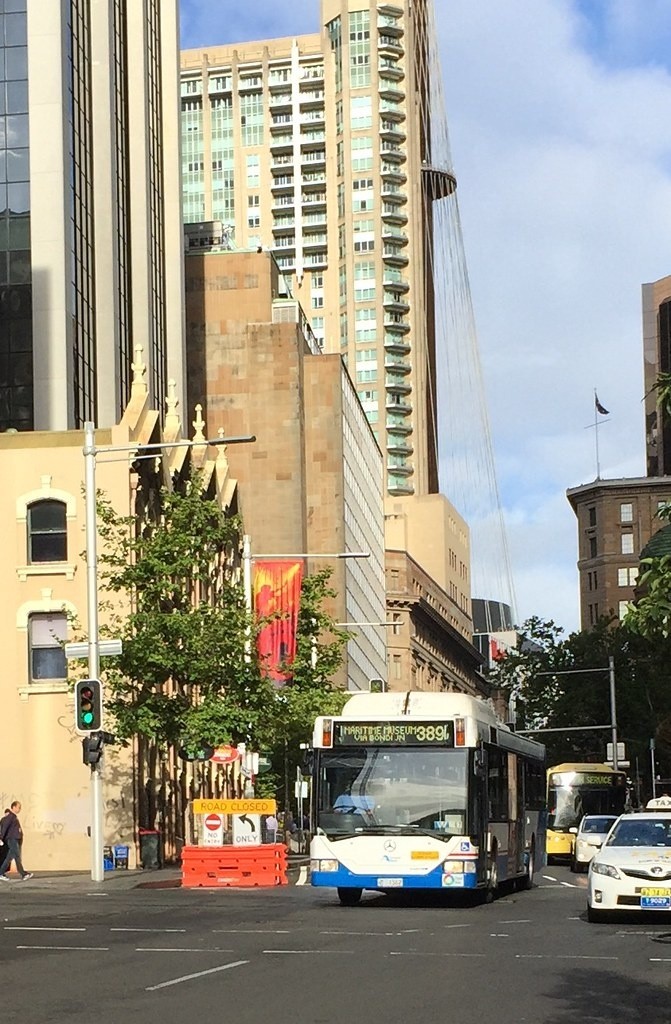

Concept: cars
[[586, 795, 671, 916], [569, 813, 620, 873]]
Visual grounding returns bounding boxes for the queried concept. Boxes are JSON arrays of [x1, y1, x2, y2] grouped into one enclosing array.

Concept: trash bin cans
[[139, 830, 165, 869]]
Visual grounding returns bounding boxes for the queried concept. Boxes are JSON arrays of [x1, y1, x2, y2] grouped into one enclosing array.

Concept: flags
[[596, 395, 609, 416]]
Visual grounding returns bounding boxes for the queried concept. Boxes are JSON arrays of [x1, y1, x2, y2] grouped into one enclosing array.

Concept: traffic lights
[[369, 677, 385, 692], [82, 738, 101, 765], [75, 679, 102, 733]]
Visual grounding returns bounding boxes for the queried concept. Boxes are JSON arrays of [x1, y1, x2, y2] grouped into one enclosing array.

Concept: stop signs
[[206, 813, 222, 831]]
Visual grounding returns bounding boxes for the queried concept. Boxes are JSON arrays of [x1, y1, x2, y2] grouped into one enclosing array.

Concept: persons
[[613, 825, 657, 845], [0, 801, 34, 881], [333, 776, 375, 815], [551, 803, 557, 814], [266, 814, 278, 844], [477, 783, 507, 819], [287, 809, 311, 853], [591, 823, 599, 831]]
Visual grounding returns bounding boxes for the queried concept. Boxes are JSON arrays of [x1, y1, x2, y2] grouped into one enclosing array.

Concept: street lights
[[85, 419, 257, 880]]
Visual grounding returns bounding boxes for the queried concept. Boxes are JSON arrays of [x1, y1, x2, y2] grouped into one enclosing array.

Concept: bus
[[542, 762, 636, 867], [298, 690, 550, 904]]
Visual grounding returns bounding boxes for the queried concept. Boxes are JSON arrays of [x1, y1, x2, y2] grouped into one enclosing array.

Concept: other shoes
[[0, 875, 9, 881], [22, 872, 33, 881]]
